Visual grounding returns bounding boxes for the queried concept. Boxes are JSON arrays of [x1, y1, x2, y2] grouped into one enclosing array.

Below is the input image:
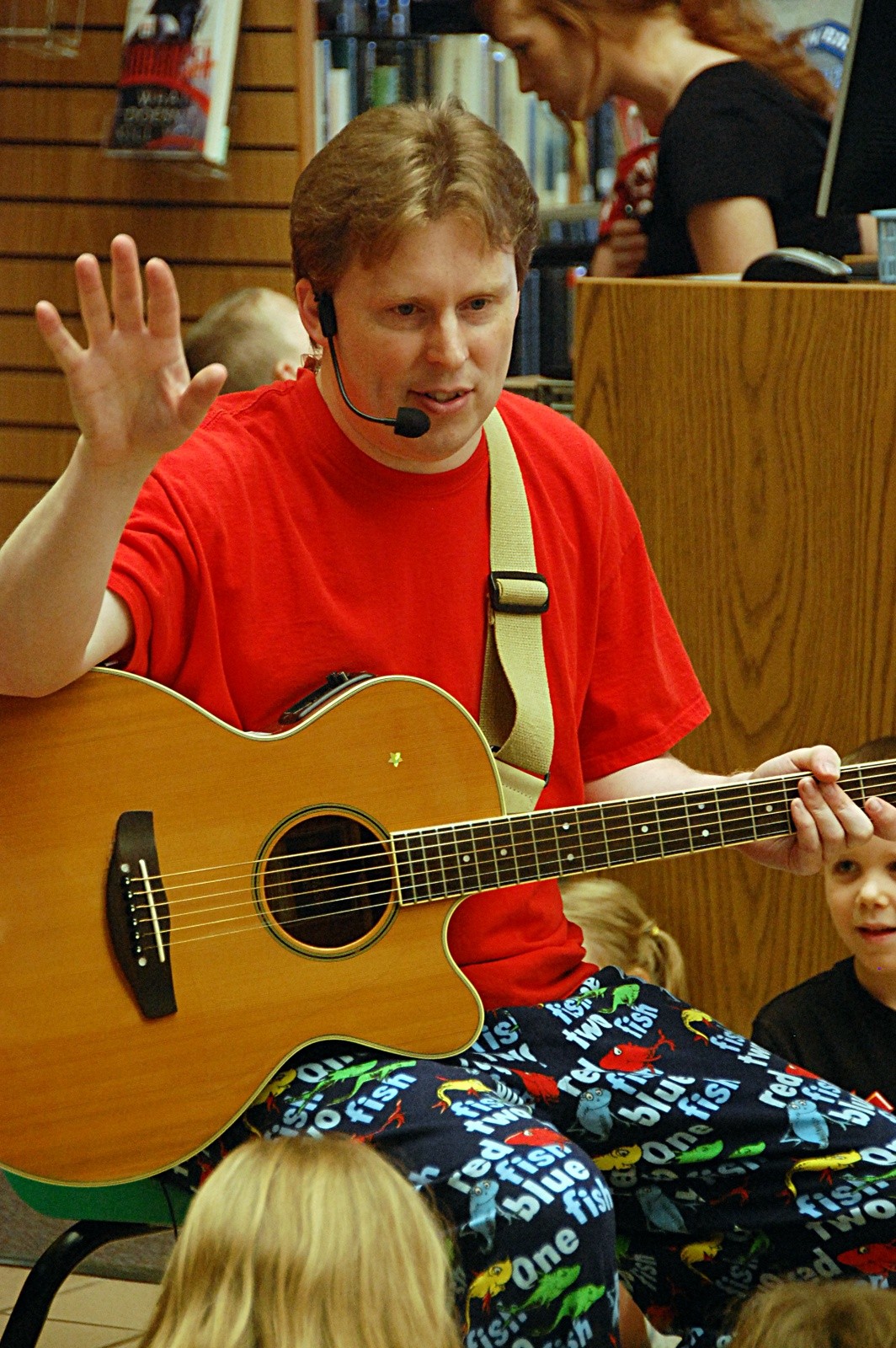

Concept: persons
[[560, 875, 689, 1348], [474, 0, 878, 276], [753, 833, 896, 1115], [138, 1137, 461, 1348], [180, 289, 314, 397], [725, 1283, 896, 1348], [0, 99, 896, 1348]]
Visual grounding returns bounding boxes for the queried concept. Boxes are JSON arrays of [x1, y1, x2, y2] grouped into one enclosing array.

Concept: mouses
[[740, 247, 853, 287]]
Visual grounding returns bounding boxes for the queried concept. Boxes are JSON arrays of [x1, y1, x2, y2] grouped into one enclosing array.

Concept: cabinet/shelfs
[[312, 2, 682, 423]]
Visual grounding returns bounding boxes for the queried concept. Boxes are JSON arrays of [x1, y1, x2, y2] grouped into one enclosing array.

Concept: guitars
[[0, 656, 896, 1190]]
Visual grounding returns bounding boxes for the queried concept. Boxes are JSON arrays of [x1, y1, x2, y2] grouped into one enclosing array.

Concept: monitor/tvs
[[815, 0, 896, 215]]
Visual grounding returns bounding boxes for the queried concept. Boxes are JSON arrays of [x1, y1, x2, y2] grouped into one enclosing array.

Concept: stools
[[0, 1166, 218, 1348]]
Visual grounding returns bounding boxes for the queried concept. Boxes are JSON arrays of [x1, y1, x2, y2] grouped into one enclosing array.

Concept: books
[[308, 1, 655, 243], [104, 0, 243, 165], [508, 264, 579, 380]]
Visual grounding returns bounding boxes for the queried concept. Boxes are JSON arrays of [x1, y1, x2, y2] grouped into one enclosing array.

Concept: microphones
[[326, 335, 433, 440]]
